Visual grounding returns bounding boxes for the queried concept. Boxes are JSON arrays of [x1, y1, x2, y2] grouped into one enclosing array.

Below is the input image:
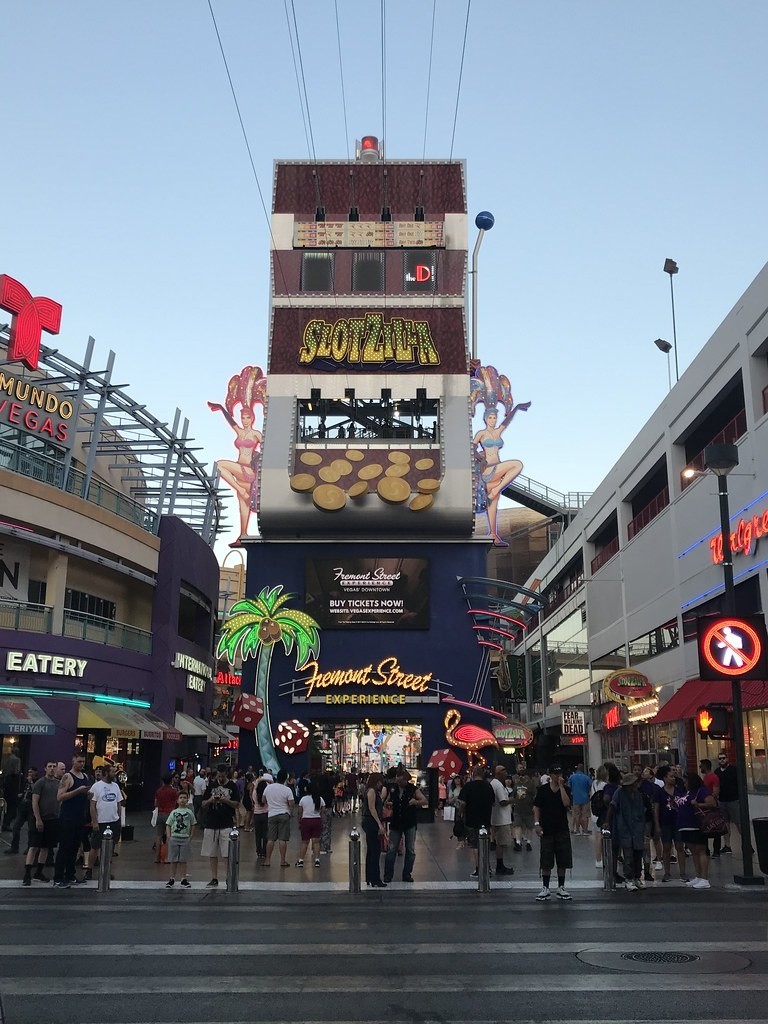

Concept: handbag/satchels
[[453, 821, 464, 837], [702, 812, 728, 838], [151, 807, 158, 827], [443, 807, 455, 822], [337, 787, 343, 797], [379, 834, 388, 852]]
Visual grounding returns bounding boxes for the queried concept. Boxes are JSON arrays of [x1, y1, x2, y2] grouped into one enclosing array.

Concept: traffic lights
[[697, 613, 768, 681], [696, 706, 730, 735]]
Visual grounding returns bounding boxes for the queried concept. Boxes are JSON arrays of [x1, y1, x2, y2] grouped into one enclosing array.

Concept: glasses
[[718, 757, 727, 760], [519, 774, 525, 776]]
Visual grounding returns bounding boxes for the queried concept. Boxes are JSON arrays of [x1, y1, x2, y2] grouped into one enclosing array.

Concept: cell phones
[[214, 796, 220, 799]]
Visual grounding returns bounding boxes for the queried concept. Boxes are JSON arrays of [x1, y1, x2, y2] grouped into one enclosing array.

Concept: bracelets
[[417, 800, 418, 806]]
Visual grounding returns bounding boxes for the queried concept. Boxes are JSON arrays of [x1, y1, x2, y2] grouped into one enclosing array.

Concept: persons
[[201, 766, 241, 887], [295, 784, 326, 867], [435, 753, 755, 859], [252, 778, 274, 859], [676, 771, 718, 888], [458, 767, 495, 878], [166, 791, 197, 888], [603, 773, 652, 892], [152, 763, 404, 863], [534, 765, 574, 900], [361, 773, 387, 887], [261, 770, 294, 867], [0, 752, 124, 888], [383, 770, 428, 882], [491, 766, 516, 875]]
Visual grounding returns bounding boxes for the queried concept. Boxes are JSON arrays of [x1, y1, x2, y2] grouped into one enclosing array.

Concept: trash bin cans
[[753, 817, 768, 876]]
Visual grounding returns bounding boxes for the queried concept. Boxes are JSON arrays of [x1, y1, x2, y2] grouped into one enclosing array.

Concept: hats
[[622, 773, 638, 785], [450, 772, 459, 780], [589, 768, 595, 771], [549, 766, 567, 774]]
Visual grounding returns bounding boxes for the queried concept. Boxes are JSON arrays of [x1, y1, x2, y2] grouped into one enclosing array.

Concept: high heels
[[366, 879, 387, 887]]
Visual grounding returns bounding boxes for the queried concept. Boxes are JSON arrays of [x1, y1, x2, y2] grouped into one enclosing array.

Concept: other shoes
[[256, 850, 332, 868], [453, 833, 536, 881], [3, 843, 227, 889], [383, 878, 391, 882], [405, 877, 414, 883]]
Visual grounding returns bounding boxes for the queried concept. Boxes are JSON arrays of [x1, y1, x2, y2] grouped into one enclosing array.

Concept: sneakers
[[536, 844, 735, 900]]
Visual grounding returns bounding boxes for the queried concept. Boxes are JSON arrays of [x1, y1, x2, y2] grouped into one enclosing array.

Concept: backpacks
[[591, 784, 609, 817]]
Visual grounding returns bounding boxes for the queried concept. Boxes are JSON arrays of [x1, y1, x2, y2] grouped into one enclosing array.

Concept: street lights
[[703, 442, 764, 886]]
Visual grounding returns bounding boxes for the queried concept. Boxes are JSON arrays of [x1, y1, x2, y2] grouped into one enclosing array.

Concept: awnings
[[0, 696, 235, 744]]
